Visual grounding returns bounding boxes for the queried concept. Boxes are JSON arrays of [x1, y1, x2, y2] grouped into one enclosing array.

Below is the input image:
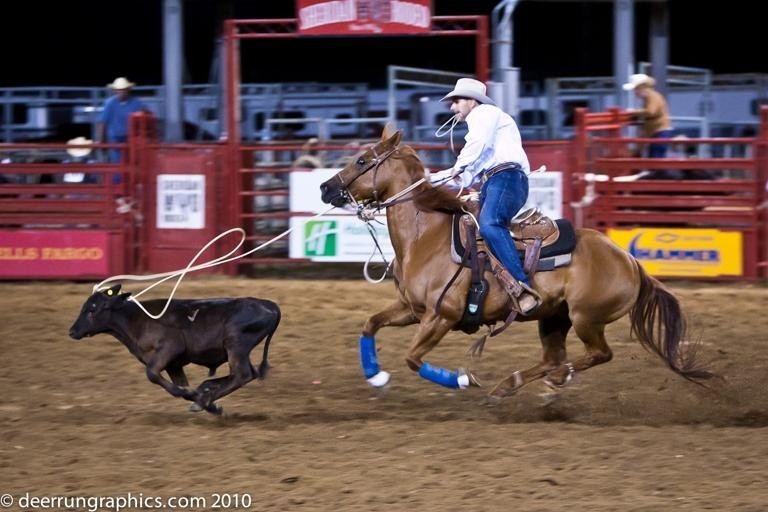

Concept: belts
[[479, 162, 524, 183]]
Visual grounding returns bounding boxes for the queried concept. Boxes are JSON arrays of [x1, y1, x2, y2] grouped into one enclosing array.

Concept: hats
[[439, 77, 497, 107], [65, 137, 95, 159], [107, 76, 136, 91], [622, 73, 655, 91]]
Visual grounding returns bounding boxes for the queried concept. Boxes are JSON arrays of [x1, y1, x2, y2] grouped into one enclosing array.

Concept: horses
[[319, 121, 726, 408]]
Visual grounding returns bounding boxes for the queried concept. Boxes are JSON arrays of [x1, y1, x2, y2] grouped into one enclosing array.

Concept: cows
[[68, 284, 281, 415]]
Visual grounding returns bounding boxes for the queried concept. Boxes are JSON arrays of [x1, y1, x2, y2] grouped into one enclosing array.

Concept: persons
[[436, 77, 543, 316], [664, 143, 689, 179], [93, 76, 151, 182], [620, 73, 674, 180], [48, 136, 105, 227]]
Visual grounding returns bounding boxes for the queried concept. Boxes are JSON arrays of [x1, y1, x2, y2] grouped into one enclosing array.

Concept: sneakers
[[514, 291, 540, 312]]
